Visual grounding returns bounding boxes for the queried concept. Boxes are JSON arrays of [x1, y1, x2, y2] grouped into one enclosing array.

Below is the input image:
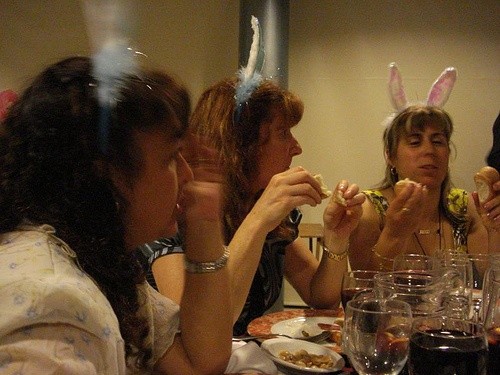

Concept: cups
[[339, 249, 500, 375]]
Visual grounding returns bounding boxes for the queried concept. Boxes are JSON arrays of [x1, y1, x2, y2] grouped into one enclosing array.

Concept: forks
[[232, 331, 330, 341]]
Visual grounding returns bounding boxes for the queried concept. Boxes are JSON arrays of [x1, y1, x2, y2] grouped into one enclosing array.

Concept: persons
[[1, 1, 230, 375], [348, 61, 500, 299], [141, 15, 365, 328]]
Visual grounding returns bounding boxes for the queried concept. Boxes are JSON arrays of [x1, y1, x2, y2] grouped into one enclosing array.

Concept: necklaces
[[413, 210, 442, 256]]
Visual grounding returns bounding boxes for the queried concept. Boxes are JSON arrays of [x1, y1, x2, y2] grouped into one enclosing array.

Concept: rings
[[402, 207, 411, 213]]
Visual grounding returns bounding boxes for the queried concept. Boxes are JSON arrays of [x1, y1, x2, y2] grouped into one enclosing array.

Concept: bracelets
[[183, 247, 231, 273], [323, 245, 347, 261]]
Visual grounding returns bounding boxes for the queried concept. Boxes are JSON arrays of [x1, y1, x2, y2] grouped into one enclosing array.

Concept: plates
[[270, 317, 345, 353], [260, 339, 345, 375]]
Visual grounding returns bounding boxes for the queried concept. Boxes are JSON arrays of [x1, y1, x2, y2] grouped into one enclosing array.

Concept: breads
[[310, 174, 332, 200], [394, 178, 418, 198], [474, 166, 500, 232], [334, 191, 347, 206]]
